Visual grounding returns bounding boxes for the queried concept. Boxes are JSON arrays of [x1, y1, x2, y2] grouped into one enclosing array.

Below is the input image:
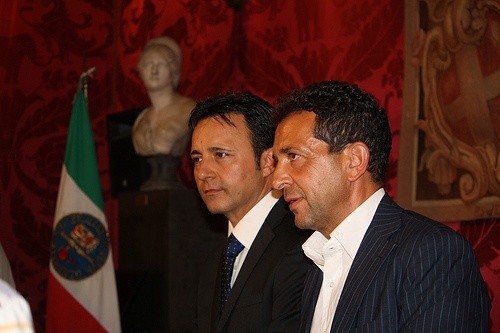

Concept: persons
[[189, 90, 316, 333], [129, 36, 201, 192], [273, 80, 492, 333]]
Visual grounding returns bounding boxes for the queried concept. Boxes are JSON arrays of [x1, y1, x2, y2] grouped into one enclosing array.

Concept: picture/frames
[[396, 0, 500, 222]]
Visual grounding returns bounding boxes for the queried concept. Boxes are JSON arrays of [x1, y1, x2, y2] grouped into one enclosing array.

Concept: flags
[[44, 85, 123, 333]]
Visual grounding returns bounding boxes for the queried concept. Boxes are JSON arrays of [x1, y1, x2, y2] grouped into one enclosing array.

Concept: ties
[[218, 234, 245, 312]]
